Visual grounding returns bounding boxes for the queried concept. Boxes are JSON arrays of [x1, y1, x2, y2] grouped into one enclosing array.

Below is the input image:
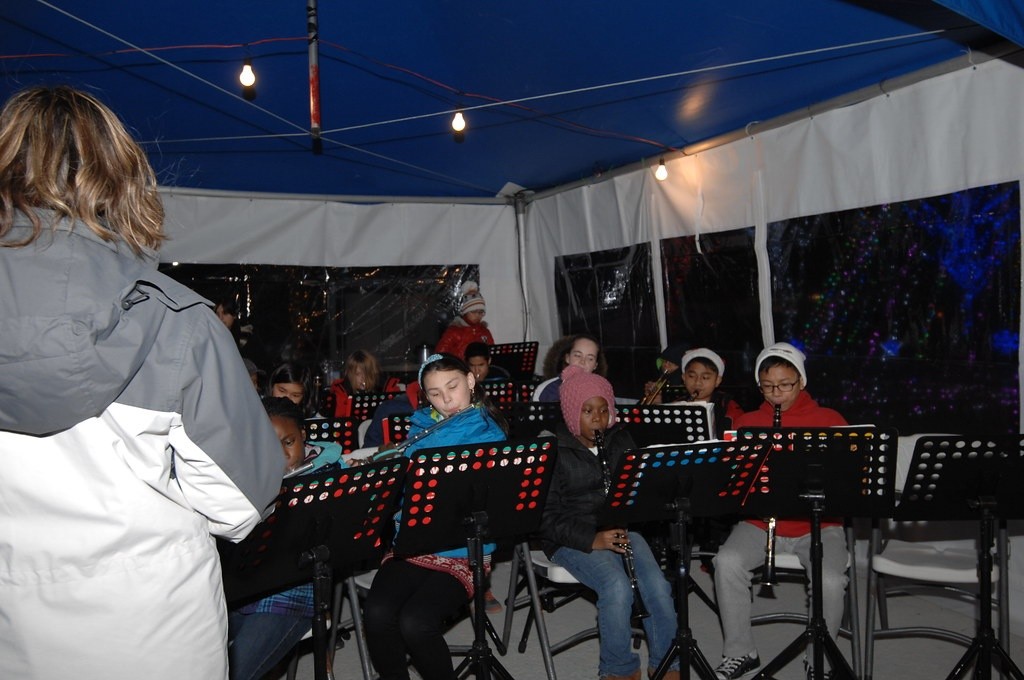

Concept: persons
[[209, 283, 848, 680], [1, 86, 286, 680]]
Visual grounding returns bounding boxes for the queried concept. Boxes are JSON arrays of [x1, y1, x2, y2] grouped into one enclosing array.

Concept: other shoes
[[484, 591, 502, 613], [599, 668, 679, 680]]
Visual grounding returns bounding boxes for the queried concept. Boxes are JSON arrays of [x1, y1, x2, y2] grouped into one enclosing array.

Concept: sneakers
[[802, 654, 830, 680], [714, 648, 762, 680]]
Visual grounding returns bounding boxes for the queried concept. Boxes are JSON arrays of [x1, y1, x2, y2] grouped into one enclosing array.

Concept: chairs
[[288, 378, 1010, 680]]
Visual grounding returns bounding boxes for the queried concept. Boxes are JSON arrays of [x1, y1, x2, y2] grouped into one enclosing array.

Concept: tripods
[[893, 433, 1024, 680], [597, 438, 774, 680], [393, 435, 558, 680], [736, 425, 899, 679]]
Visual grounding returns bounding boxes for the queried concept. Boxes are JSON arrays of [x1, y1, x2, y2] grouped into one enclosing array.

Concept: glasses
[[468, 310, 486, 316], [755, 342, 807, 386], [661, 359, 674, 365], [756, 379, 799, 394]]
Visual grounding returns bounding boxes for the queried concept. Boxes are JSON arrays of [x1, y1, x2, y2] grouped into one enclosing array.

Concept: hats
[[461, 281, 486, 316], [657, 344, 684, 365], [242, 358, 265, 378], [559, 364, 616, 437], [682, 348, 725, 376]]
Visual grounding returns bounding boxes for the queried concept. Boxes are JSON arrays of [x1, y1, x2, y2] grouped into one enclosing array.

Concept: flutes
[[394, 403, 476, 451], [758, 403, 782, 588], [594, 428, 652, 621], [281, 462, 316, 479]]
